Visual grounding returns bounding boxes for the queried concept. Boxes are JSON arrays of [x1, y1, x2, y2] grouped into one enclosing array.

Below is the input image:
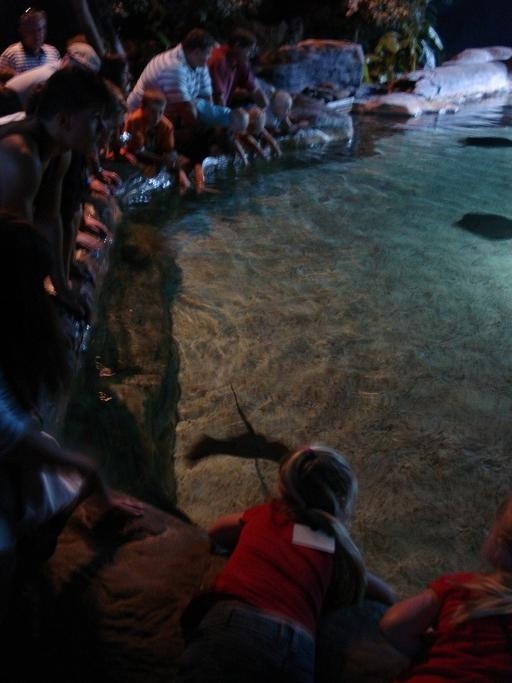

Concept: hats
[[67, 42, 102, 73]]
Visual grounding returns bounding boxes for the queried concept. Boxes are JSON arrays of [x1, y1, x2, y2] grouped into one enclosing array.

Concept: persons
[[378, 496, 512, 682], [170, 445, 396, 682], [1, 7, 309, 551]]
